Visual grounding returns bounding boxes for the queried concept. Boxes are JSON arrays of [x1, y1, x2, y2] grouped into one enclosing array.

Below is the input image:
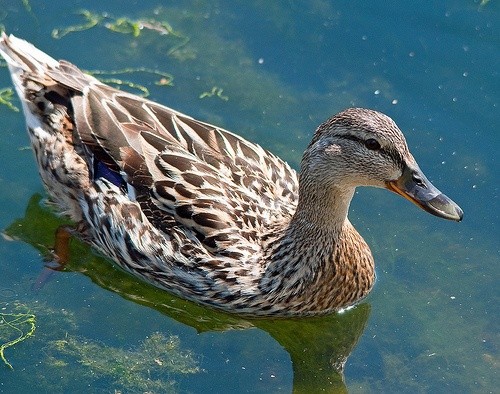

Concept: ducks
[[0, 30, 466, 320]]
